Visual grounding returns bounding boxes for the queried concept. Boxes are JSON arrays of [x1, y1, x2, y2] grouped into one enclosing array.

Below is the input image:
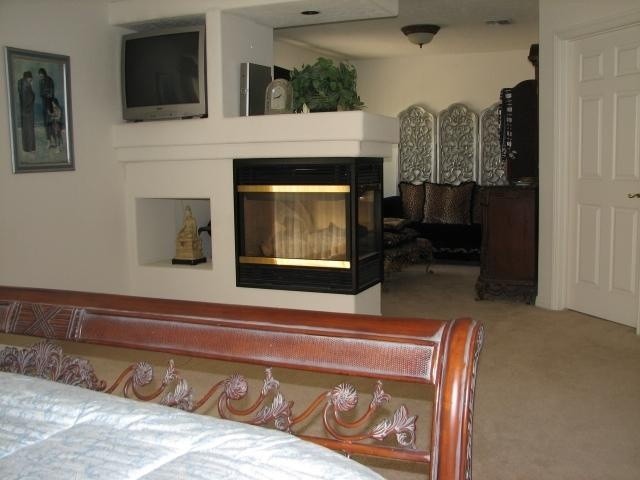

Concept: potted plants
[[291, 58, 363, 112]]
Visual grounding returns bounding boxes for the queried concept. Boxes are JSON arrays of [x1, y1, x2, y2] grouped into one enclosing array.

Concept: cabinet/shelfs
[[480, 42, 538, 303]]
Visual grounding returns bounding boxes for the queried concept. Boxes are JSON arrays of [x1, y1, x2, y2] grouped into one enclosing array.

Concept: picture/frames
[[4, 45, 76, 175]]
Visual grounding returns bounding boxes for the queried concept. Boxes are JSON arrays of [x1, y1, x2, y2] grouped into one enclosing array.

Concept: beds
[[0, 284, 484, 480]]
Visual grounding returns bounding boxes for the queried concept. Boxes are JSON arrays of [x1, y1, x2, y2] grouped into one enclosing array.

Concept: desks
[[384, 236, 435, 273]]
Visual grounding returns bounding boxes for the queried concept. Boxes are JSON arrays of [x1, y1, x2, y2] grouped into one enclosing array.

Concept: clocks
[[266, 78, 291, 115]]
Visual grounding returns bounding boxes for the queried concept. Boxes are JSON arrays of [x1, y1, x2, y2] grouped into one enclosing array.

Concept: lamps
[[401, 24, 441, 48]]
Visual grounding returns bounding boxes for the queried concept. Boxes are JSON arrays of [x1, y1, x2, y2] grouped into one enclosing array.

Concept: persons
[[37, 66, 56, 151], [173, 204, 202, 243], [17, 69, 38, 153], [44, 97, 65, 156]]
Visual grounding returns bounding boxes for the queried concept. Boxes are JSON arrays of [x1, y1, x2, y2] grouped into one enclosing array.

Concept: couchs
[[378, 180, 482, 262]]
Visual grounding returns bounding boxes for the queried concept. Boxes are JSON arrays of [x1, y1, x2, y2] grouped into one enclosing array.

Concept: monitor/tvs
[[120, 24, 208, 121]]
